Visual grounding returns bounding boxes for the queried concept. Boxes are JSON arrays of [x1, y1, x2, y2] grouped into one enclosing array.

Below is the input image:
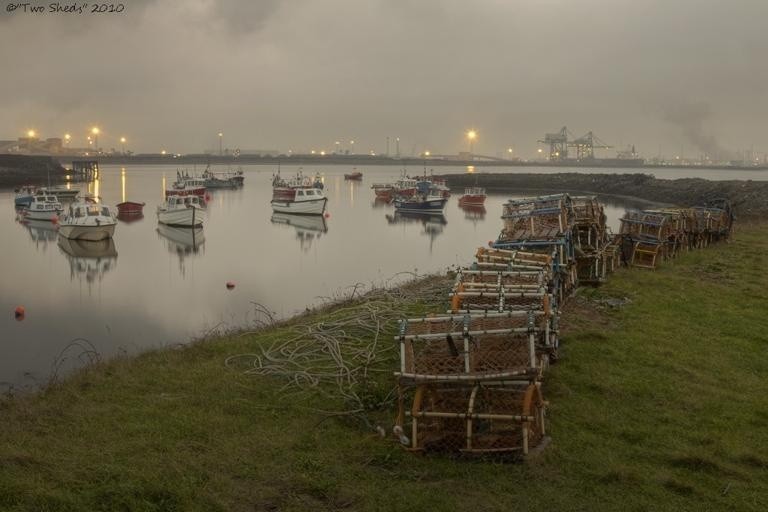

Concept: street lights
[[467, 130, 477, 161], [92, 127, 99, 152], [119, 137, 126, 156], [216, 128, 225, 155]]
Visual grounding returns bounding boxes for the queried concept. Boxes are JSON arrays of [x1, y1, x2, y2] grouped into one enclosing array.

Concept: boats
[[57, 236, 118, 308], [15, 183, 145, 241], [270, 212, 328, 253], [370, 167, 487, 215], [156, 163, 246, 226], [155, 225, 206, 279], [344, 171, 363, 179], [270, 167, 328, 214]]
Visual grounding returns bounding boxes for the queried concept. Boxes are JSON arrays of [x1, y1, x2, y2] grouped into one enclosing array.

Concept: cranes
[[538, 126, 615, 162]]
